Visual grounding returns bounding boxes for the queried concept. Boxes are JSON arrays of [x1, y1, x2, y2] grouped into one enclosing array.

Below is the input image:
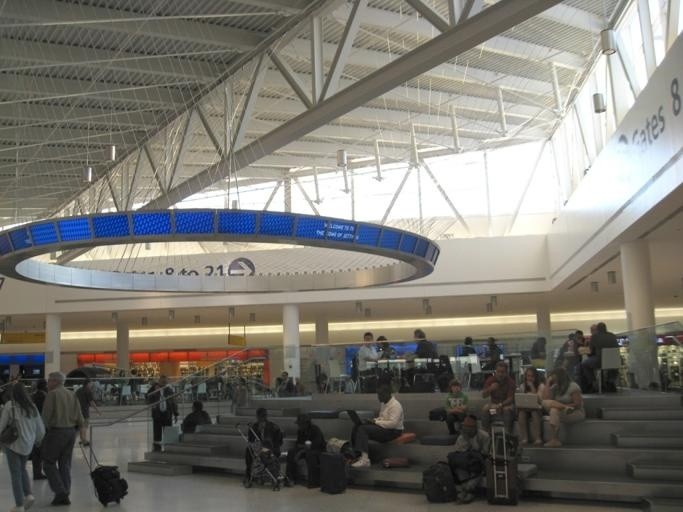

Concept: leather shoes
[[34, 475, 48, 479], [61, 495, 69, 505], [52, 493, 67, 504]]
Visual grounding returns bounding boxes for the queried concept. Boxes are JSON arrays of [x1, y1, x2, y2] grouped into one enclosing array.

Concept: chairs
[[72, 376, 208, 401]]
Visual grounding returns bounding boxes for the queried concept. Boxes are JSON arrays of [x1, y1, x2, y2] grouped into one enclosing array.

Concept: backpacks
[[422, 461, 457, 503]]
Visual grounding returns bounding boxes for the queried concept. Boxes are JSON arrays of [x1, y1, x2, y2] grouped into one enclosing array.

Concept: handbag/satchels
[[447, 451, 484, 484], [0, 422, 19, 444]]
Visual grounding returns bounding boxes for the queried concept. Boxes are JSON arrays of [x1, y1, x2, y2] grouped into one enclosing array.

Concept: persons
[[144, 376, 178, 451], [179, 400, 212, 431], [0, 370, 96, 511], [235, 321, 627, 505], [115, 365, 236, 409]]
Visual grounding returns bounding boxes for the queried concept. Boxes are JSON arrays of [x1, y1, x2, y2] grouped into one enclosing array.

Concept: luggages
[[78, 439, 128, 506], [319, 451, 346, 494], [485, 421, 521, 505]]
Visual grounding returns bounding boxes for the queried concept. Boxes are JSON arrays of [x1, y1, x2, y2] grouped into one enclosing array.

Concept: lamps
[[593, 0, 618, 114], [110, 307, 257, 327], [336, 115, 346, 167], [82, 108, 115, 183]]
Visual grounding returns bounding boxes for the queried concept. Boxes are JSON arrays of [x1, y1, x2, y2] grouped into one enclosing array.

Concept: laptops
[[347, 409, 369, 425], [514, 393, 542, 410]]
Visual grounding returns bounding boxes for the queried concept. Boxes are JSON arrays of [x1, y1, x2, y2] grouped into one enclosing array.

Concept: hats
[[295, 414, 312, 424]]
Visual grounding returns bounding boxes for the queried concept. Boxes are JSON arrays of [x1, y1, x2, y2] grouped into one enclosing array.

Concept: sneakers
[[564, 406, 575, 414], [458, 490, 474, 502], [351, 457, 371, 468], [543, 440, 562, 447], [12, 506, 25, 511], [26, 495, 35, 508]]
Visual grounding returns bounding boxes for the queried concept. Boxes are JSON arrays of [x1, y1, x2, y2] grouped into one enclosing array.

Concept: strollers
[[234, 418, 293, 493]]
